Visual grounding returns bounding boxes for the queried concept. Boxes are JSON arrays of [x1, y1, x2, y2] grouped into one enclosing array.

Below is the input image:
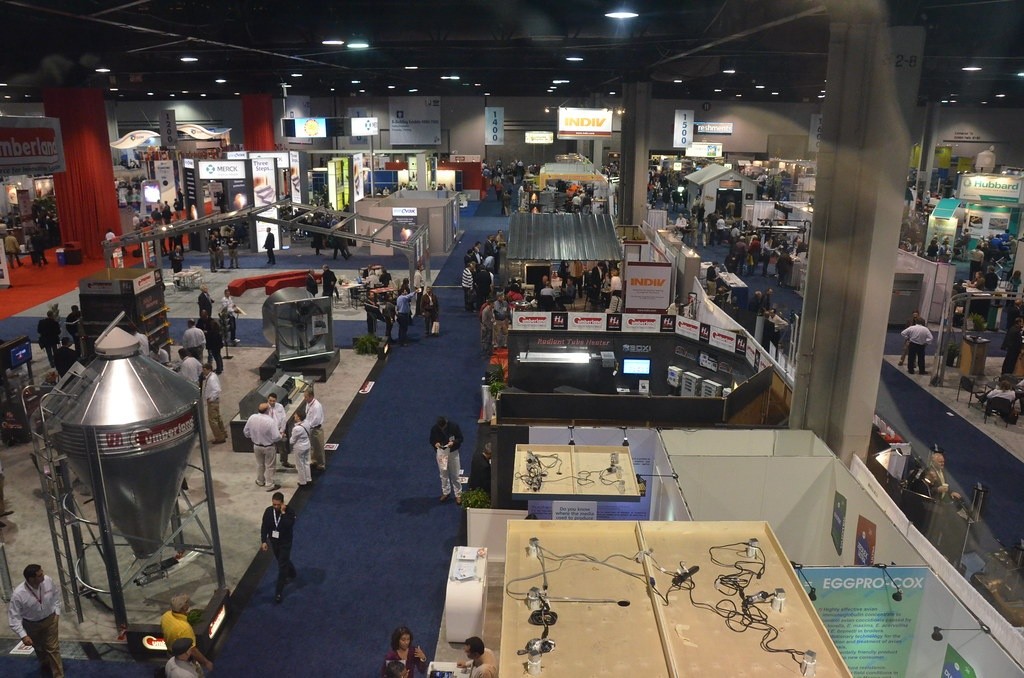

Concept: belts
[[256, 444, 273, 447]]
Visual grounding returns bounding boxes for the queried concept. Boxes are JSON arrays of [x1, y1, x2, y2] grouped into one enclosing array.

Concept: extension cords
[[672, 565, 699, 584]]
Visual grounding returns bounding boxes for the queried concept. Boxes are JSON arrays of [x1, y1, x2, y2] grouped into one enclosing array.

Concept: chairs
[[189, 265, 204, 289], [984, 396, 1015, 428], [583, 287, 605, 313], [957, 376, 987, 408], [164, 281, 177, 296]]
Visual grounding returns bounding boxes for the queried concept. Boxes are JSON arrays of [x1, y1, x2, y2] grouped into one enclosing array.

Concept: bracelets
[[421, 657, 427, 662]]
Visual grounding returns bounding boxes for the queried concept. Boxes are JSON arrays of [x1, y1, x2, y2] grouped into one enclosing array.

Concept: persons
[[8, 564, 65, 678], [261, 491, 297, 603], [468, 443, 491, 496], [166, 638, 199, 678], [456, 637, 497, 678], [0, 148, 440, 528], [381, 627, 428, 678], [385, 661, 409, 678], [429, 416, 464, 504], [161, 594, 212, 678], [460, 156, 1024, 415]]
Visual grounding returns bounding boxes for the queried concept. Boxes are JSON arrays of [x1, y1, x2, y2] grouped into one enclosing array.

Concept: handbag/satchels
[[431, 321, 441, 334], [467, 289, 478, 303], [747, 255, 753, 266]]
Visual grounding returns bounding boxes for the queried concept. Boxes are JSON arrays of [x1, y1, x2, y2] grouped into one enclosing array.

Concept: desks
[[717, 273, 749, 310], [426, 661, 471, 678], [787, 261, 803, 287], [173, 271, 199, 290], [986, 380, 999, 389], [970, 294, 992, 323], [445, 546, 488, 643]]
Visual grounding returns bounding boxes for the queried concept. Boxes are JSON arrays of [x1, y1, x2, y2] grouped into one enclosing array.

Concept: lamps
[[553, 65, 571, 84], [622, 429, 630, 446], [961, 59, 983, 71], [882, 567, 904, 602], [636, 474, 673, 484], [321, 32, 345, 45], [791, 562, 817, 602], [565, 49, 583, 61], [605, 0, 640, 19], [931, 626, 983, 641], [568, 428, 575, 445]]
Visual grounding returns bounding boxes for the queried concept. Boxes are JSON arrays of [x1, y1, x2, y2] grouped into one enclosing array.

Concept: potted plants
[[487, 380, 509, 411], [943, 338, 961, 367], [457, 487, 495, 546]]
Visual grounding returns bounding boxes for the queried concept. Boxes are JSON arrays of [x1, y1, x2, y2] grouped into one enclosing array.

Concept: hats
[[172, 638, 194, 655]]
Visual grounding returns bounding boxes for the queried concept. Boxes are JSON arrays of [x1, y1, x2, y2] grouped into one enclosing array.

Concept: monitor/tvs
[[623, 359, 650, 374], [10, 340, 32, 370]]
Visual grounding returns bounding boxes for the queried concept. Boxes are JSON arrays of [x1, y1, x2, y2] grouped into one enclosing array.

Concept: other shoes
[[282, 462, 292, 468], [898, 360, 904, 366], [440, 494, 449, 501], [456, 497, 461, 504], [211, 435, 228, 444], [266, 485, 281, 492], [297, 481, 313, 487], [255, 479, 265, 486], [975, 393, 982, 403], [233, 338, 240, 342], [315, 467, 326, 473], [275, 592, 283, 602]]
